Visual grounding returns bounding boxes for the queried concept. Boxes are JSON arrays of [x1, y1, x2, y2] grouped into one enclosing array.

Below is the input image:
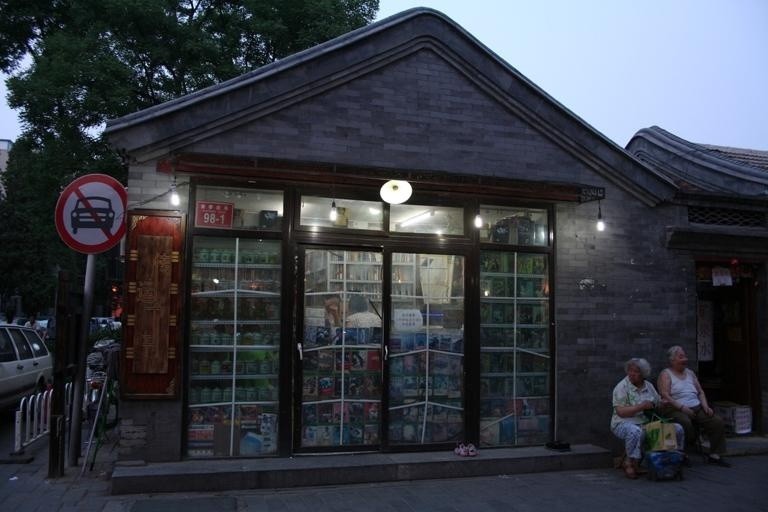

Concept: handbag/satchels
[[643, 409, 677, 453]]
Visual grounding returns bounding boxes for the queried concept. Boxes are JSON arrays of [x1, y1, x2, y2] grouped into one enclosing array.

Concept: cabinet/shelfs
[[479, 249, 550, 420], [302, 248, 418, 305], [189, 228, 285, 428]]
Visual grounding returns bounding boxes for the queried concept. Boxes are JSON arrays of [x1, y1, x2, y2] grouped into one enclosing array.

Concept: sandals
[[468, 444, 477, 457], [454, 444, 469, 456]]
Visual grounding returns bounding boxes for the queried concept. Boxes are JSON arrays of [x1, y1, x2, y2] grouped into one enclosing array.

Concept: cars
[[0, 312, 120, 404]]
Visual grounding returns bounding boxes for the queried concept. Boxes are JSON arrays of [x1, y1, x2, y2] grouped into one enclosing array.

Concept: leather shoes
[[621, 460, 637, 479], [708, 454, 732, 468]]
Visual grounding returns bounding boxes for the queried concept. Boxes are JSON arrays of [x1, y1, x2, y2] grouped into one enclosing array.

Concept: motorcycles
[[87, 337, 117, 444]]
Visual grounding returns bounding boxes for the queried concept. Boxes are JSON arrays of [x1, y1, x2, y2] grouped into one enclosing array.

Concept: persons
[[610, 358, 685, 479], [2, 312, 16, 324], [24, 314, 42, 338], [345, 296, 382, 328], [657, 345, 732, 467]]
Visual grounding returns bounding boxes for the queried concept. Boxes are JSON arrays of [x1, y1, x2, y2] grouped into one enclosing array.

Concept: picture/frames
[[118, 207, 188, 402]]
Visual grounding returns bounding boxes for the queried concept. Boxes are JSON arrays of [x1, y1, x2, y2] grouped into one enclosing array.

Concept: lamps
[[330, 197, 337, 221], [474, 196, 483, 228], [170, 178, 180, 206], [595, 199, 607, 232], [379, 179, 413, 206]]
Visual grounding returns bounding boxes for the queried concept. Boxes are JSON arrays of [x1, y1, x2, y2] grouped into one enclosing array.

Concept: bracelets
[[679, 404, 684, 412]]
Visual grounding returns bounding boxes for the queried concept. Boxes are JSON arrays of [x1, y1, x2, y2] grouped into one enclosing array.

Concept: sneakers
[[543, 440, 572, 453]]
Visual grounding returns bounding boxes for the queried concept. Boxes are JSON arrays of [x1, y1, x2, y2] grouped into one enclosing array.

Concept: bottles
[[187, 245, 279, 404], [305, 250, 414, 299]]
